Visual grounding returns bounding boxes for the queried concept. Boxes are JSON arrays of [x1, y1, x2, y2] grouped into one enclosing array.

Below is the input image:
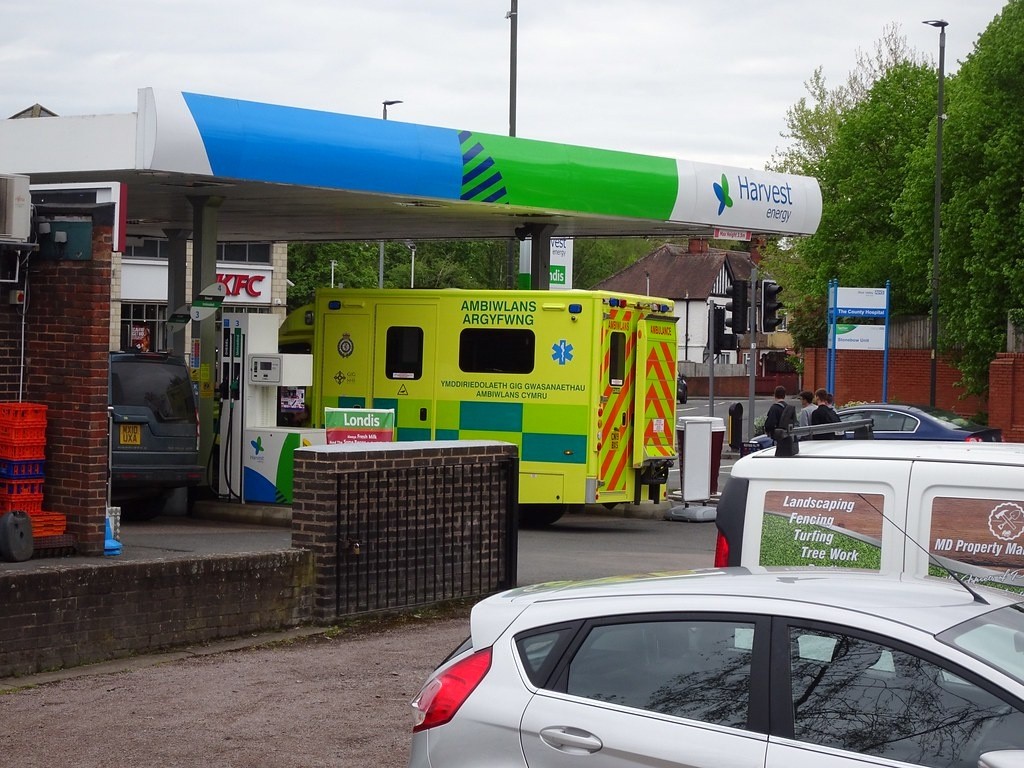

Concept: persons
[[825, 393, 838, 414], [811, 387, 842, 440], [764, 386, 795, 446], [798, 390, 819, 441]]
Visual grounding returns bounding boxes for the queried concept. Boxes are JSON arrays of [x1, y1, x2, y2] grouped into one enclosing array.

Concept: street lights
[[923, 20, 948, 406]]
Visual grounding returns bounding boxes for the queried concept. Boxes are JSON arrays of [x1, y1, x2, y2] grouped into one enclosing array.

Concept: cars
[[748, 403, 1001, 443], [675, 370, 688, 403], [405, 486, 1024, 767]]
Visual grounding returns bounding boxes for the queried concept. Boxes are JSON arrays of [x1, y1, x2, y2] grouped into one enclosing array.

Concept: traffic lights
[[724, 281, 748, 334], [760, 278, 784, 336]]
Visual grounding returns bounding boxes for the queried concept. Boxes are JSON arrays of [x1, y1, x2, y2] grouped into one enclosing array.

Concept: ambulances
[[274, 286, 680, 528]]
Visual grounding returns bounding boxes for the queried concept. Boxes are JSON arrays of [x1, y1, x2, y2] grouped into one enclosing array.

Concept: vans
[[706, 434, 1024, 603], [103, 347, 205, 517]]
